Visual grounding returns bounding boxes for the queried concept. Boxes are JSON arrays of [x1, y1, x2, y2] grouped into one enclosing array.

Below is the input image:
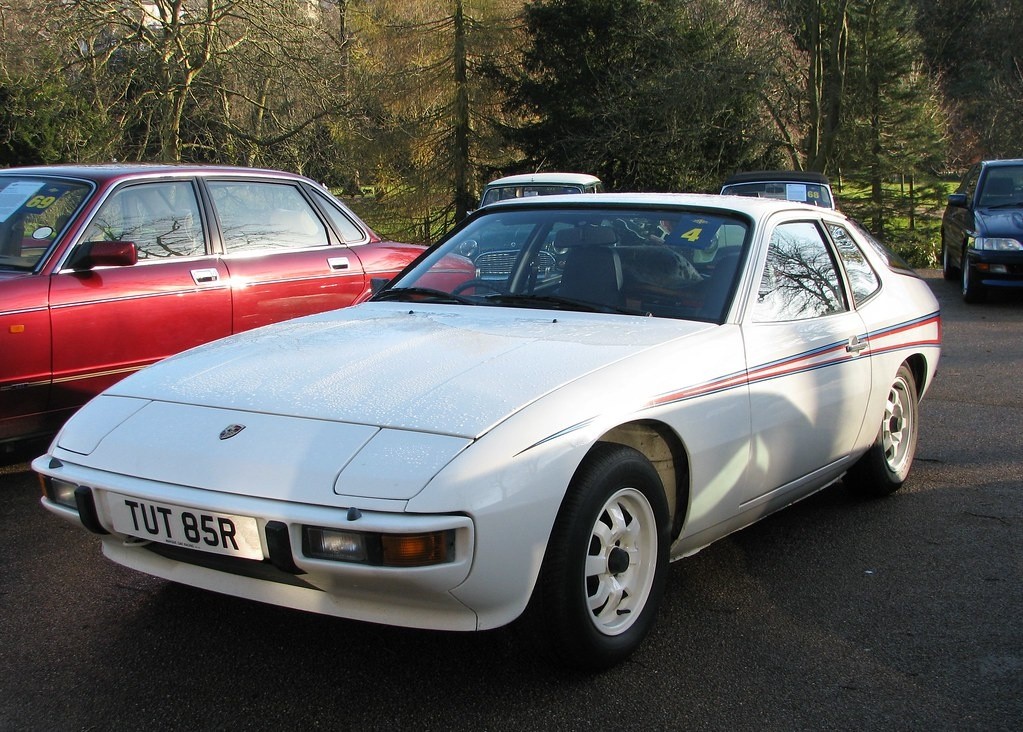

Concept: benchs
[[223, 206, 325, 252]]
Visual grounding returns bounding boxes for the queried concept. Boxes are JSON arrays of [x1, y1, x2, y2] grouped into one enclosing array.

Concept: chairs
[[696, 254, 776, 320], [980, 177, 1016, 206], [556, 245, 623, 310]]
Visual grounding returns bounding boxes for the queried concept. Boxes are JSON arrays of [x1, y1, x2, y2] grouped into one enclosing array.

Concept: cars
[[33, 194, 942, 672], [940, 159, 1023, 303], [457, 173, 606, 292], [717, 171, 838, 247], [0, 166, 480, 454]]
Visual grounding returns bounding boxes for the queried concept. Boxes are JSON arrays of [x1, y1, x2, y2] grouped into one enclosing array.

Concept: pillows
[[635, 238, 703, 289]]
